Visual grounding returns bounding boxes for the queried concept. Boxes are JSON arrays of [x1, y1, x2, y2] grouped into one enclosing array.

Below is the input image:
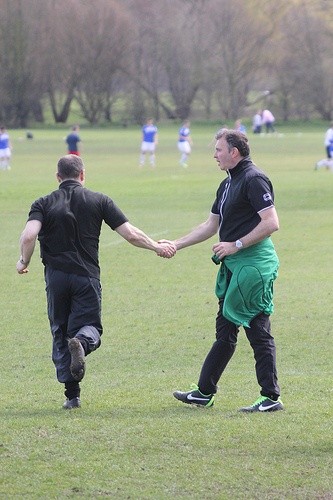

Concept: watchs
[[236, 241, 243, 248]]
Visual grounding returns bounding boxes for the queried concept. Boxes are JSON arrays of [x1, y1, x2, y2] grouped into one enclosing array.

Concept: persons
[[16, 155, 176, 409], [314, 123, 333, 170], [234, 120, 246, 132], [0, 126, 11, 170], [177, 120, 192, 166], [252, 107, 276, 134], [65, 125, 82, 156], [139, 119, 158, 169], [158, 129, 285, 413]]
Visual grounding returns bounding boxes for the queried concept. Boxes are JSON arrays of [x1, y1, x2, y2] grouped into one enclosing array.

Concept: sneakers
[[172, 383, 215, 407], [239, 395, 284, 413]]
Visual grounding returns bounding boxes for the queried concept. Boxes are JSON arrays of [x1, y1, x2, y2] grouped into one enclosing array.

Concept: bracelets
[[19, 256, 30, 264]]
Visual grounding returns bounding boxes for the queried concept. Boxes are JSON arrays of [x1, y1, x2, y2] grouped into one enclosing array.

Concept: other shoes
[[62, 397, 80, 409], [67, 337, 86, 382]]
